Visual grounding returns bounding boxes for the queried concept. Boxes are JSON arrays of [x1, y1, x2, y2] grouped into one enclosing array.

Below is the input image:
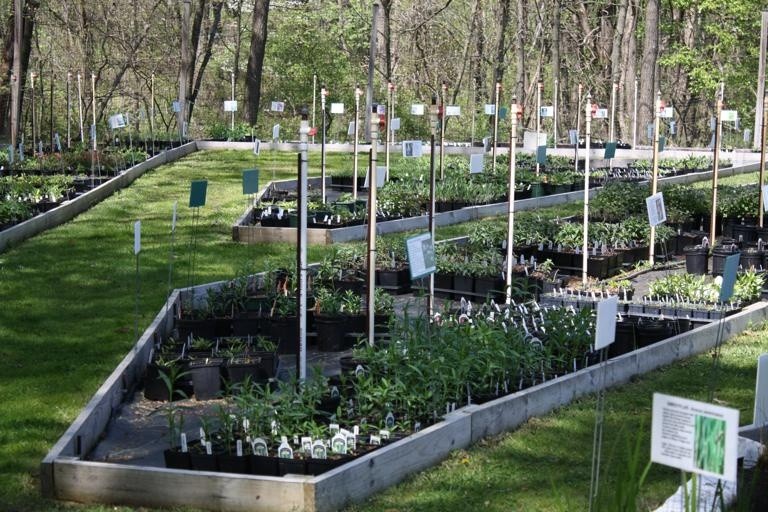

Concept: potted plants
[[0, 125, 194, 232]]
